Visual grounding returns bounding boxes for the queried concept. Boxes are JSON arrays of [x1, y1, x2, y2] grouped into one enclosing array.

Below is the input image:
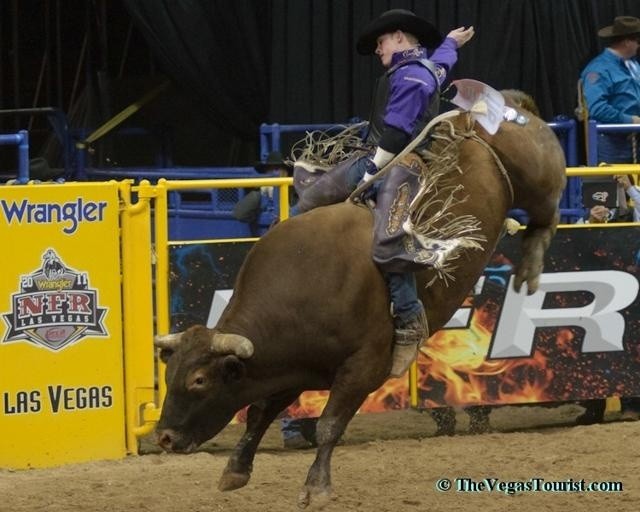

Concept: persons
[[575, 396, 640, 425], [299, 8, 475, 375], [575, 174, 640, 224], [279, 416, 348, 450], [578, 11, 640, 166], [425, 405, 494, 435], [233, 151, 300, 239]]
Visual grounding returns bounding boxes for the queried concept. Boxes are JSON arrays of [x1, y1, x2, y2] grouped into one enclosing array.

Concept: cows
[[153, 89, 567, 508]]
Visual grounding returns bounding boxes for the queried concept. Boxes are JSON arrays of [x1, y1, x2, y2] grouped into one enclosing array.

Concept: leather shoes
[[390, 322, 426, 378]]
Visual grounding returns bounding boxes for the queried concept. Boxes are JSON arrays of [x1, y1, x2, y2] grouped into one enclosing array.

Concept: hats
[[597, 15, 640, 38], [253, 152, 292, 171], [355, 8, 443, 57]]
